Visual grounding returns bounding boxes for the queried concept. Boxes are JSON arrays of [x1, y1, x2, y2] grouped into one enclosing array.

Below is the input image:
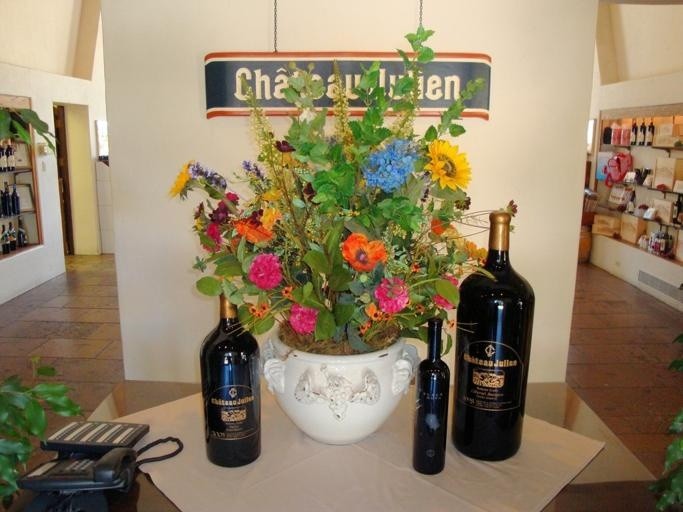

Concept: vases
[[258, 317, 421, 447]]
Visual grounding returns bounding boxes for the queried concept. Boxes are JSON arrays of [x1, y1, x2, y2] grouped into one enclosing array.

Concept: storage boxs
[[620, 212, 647, 246], [654, 122, 683, 137], [652, 158, 683, 192], [652, 136, 683, 149], [591, 213, 621, 237]]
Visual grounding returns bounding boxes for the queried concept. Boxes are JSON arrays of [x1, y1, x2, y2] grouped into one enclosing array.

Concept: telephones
[[16, 447, 137, 495]]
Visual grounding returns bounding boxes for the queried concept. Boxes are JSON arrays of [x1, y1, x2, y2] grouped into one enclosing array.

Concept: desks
[[13, 380, 664, 512]]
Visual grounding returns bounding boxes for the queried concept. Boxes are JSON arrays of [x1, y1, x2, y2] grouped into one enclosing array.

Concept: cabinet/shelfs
[[587, 104, 683, 315], [0, 95, 43, 260]]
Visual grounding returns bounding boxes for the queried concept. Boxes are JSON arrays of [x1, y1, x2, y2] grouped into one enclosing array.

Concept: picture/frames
[[7, 184, 36, 213]]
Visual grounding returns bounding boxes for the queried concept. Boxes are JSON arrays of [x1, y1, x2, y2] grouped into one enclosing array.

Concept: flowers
[[164, 24, 519, 356]]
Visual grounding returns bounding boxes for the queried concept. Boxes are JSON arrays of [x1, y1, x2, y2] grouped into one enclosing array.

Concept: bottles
[[0, 136, 27, 254], [631, 117, 655, 146], [411, 317, 450, 474], [654, 225, 669, 252], [672, 194, 683, 226], [451, 210, 535, 461], [200, 294, 261, 468]]
